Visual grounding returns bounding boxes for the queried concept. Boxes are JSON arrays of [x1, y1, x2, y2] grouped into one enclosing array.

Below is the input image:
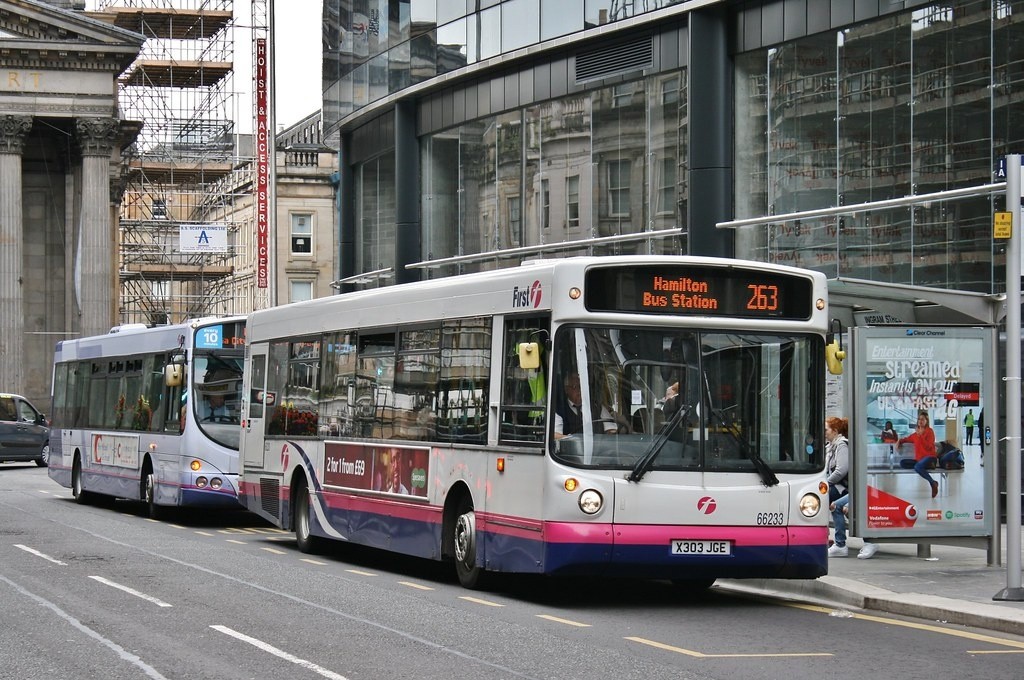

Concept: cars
[[0, 393, 50, 468]]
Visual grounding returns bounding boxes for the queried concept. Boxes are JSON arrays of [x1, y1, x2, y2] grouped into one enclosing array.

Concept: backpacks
[[937, 439, 965, 470]]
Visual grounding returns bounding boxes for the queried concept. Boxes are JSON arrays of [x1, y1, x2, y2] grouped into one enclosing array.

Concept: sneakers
[[828, 543, 848, 557], [931, 481, 939, 498], [857, 543, 880, 559]]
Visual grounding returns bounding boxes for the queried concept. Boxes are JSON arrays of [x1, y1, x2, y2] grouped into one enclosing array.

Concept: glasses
[[566, 384, 579, 389]]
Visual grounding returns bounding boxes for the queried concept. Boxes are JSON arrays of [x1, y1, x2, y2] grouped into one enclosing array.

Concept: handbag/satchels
[[831, 440, 849, 488]]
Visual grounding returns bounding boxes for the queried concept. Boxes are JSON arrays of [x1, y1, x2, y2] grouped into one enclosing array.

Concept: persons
[[553, 372, 618, 440], [881, 421, 899, 443], [896, 412, 939, 499], [978, 407, 983, 466], [827, 493, 880, 559], [197, 385, 230, 424], [964, 408, 974, 445], [825, 416, 848, 548]]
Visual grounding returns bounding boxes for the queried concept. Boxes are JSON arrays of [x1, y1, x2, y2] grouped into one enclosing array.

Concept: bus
[[238, 254, 830, 591], [47, 312, 248, 517]]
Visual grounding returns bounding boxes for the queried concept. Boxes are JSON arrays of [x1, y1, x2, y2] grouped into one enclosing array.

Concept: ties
[[575, 405, 582, 417]]
